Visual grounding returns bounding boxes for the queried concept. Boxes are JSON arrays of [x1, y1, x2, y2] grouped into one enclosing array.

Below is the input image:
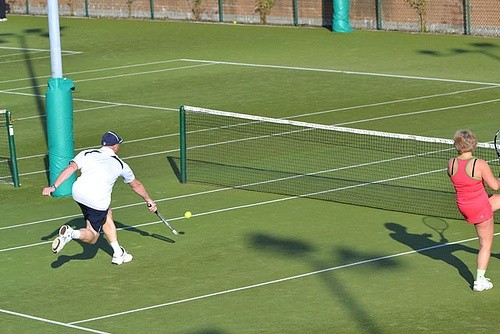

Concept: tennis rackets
[[147, 203, 179, 236], [494, 130, 500, 178]]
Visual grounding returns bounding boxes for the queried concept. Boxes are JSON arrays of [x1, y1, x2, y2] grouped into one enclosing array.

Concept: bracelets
[[51, 184, 56, 190]]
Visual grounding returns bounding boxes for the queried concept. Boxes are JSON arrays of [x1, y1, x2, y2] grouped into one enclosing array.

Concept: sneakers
[[51, 224, 72, 254], [111, 246, 133, 265], [473, 278, 494, 292]]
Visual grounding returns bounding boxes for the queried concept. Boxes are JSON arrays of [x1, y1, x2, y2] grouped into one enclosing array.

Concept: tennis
[[185, 211, 192, 218]]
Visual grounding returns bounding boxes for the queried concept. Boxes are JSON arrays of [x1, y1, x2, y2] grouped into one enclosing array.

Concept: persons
[[447, 129, 500, 292], [41, 130, 157, 265]]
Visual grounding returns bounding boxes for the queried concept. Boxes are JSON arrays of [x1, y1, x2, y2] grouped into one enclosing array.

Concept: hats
[[101, 130, 123, 146]]
